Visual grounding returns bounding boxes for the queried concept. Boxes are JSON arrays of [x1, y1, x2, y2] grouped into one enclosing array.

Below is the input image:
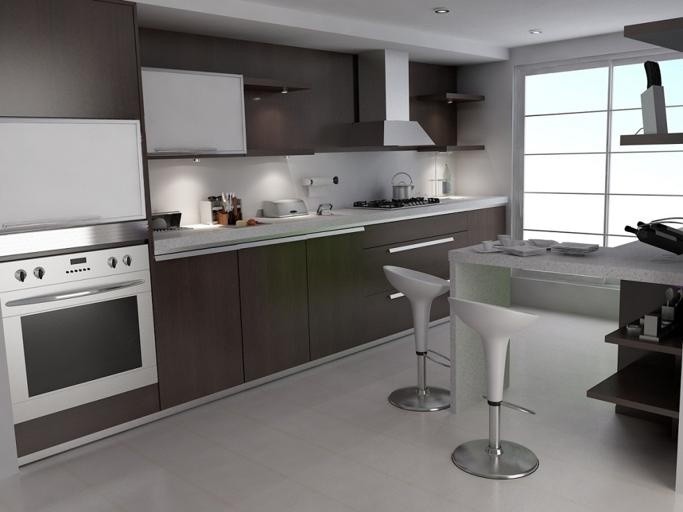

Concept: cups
[[482, 241, 492, 250], [498, 235, 511, 245]]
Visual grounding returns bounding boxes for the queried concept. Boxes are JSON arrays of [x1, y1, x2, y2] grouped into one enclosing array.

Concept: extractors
[[342, 48, 436, 147]]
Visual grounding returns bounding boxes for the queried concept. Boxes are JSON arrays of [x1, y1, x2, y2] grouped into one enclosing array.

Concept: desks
[[448, 223, 682, 493]]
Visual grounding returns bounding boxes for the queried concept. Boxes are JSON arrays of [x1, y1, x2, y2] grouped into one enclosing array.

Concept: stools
[[443, 296, 541, 478], [378, 262, 456, 413]]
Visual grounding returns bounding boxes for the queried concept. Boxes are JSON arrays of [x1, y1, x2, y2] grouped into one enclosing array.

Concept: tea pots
[[390, 171, 415, 200]]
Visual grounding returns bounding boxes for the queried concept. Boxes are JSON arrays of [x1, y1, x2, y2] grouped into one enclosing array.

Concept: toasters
[[262, 198, 308, 218]]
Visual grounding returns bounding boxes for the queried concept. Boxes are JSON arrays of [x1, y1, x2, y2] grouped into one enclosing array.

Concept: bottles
[[624, 225, 683, 256], [636, 220, 683, 236]]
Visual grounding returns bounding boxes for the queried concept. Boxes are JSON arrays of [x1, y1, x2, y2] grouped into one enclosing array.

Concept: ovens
[[0, 217, 159, 468]]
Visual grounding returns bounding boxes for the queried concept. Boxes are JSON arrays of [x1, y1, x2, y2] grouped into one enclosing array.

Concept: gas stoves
[[351, 196, 443, 211]]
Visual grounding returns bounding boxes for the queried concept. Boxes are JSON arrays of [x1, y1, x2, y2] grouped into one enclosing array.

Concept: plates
[[473, 247, 504, 254], [494, 244, 511, 249]]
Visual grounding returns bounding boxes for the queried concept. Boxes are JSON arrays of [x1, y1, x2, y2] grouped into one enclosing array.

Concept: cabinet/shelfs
[[149, 236, 244, 411], [470, 205, 507, 247], [1, 0, 145, 117], [363, 212, 470, 350], [239, 226, 363, 388], [357, 53, 485, 154], [0, 117, 152, 235]]
